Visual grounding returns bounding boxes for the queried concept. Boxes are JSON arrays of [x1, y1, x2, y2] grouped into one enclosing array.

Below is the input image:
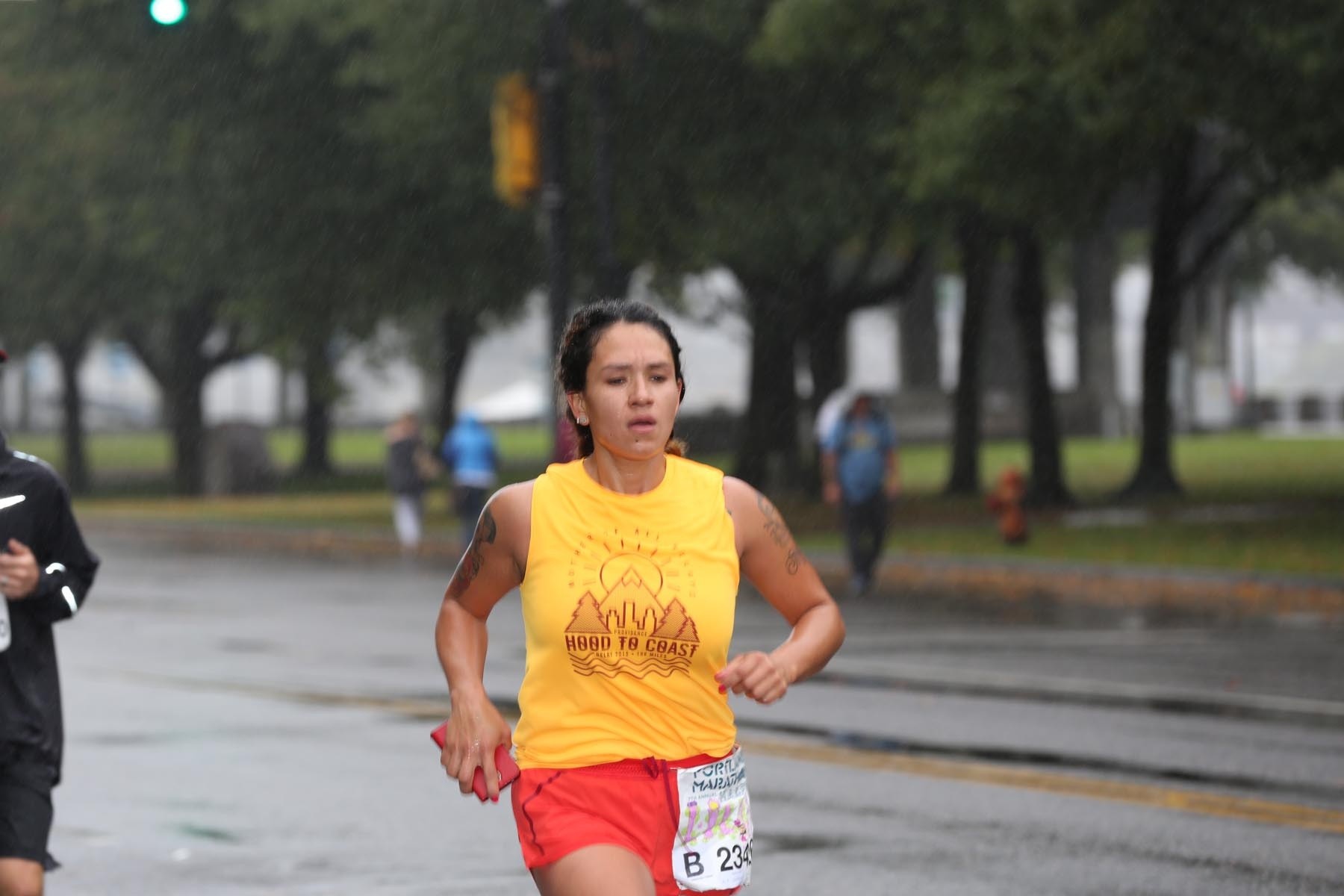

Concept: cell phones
[[430, 721, 521, 802]]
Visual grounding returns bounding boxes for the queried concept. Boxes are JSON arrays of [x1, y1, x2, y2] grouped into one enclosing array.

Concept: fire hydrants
[[982, 469, 1031, 544]]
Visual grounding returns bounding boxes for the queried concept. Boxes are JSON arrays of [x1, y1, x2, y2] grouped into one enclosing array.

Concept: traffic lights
[[492, 69, 545, 198]]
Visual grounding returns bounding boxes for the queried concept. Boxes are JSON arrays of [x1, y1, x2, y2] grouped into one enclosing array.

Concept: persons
[[385, 297, 847, 896], [0, 436, 100, 896], [821, 393, 904, 591]]
